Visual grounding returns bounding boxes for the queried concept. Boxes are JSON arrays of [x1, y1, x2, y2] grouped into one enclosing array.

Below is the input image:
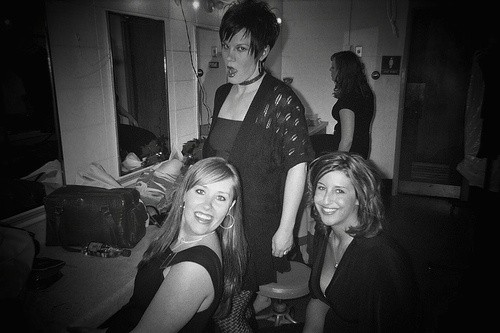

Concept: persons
[[115, 93, 160, 156], [202, 0, 315, 333], [106, 155, 248, 333], [254, 151, 432, 333], [309, 51, 375, 161]]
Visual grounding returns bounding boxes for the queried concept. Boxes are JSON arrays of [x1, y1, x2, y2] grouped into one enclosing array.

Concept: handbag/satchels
[[45, 185, 149, 249]]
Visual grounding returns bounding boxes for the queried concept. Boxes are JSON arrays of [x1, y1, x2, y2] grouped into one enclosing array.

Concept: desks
[[0, 228, 176, 333], [307, 122, 328, 137]]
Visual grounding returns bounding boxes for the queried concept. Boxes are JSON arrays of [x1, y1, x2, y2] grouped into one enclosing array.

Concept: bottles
[[81, 242, 130, 258]]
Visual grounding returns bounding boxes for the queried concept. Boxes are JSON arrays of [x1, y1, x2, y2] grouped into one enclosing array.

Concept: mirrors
[[106, 10, 171, 176], [0, 0, 66, 227]]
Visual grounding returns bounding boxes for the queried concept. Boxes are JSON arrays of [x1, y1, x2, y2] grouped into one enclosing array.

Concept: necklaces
[[171, 230, 216, 250], [239, 71, 264, 85], [332, 234, 352, 268]]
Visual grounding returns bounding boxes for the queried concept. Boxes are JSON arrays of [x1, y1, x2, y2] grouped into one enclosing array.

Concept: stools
[[257, 261, 312, 327]]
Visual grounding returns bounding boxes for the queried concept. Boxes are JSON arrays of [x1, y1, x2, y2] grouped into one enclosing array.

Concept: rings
[[284, 250, 287, 255]]
